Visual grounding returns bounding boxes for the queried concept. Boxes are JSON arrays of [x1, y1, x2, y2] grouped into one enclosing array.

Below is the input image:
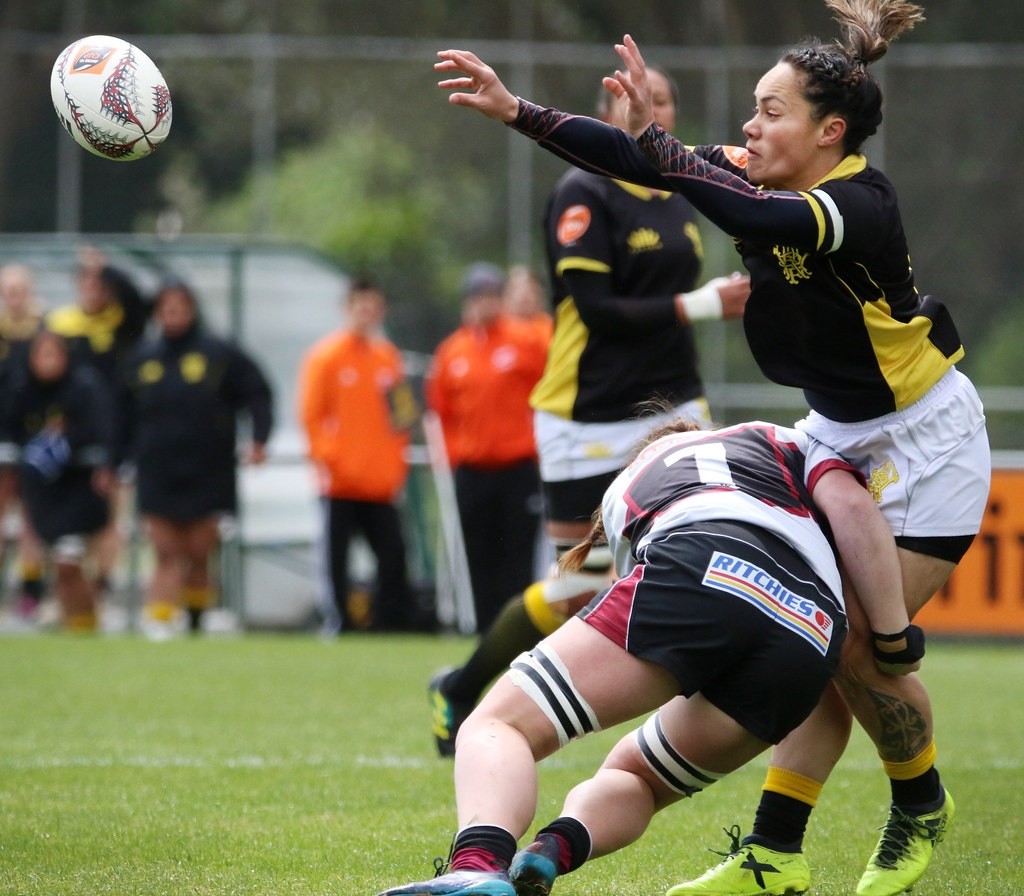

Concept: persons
[[0, 245, 274, 641], [432, 0, 992, 896], [293, 260, 554, 634]]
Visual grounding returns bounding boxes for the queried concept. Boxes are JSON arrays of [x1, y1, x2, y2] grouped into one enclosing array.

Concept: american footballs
[[49, 35, 173, 162]]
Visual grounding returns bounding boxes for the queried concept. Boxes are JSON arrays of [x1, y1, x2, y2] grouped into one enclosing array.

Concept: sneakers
[[665, 825, 810, 896], [508, 835, 558, 896], [856, 785, 955, 896], [375, 833, 517, 896]]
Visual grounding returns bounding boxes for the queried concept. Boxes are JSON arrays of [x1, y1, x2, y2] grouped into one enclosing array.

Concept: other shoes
[[428, 669, 473, 756]]
